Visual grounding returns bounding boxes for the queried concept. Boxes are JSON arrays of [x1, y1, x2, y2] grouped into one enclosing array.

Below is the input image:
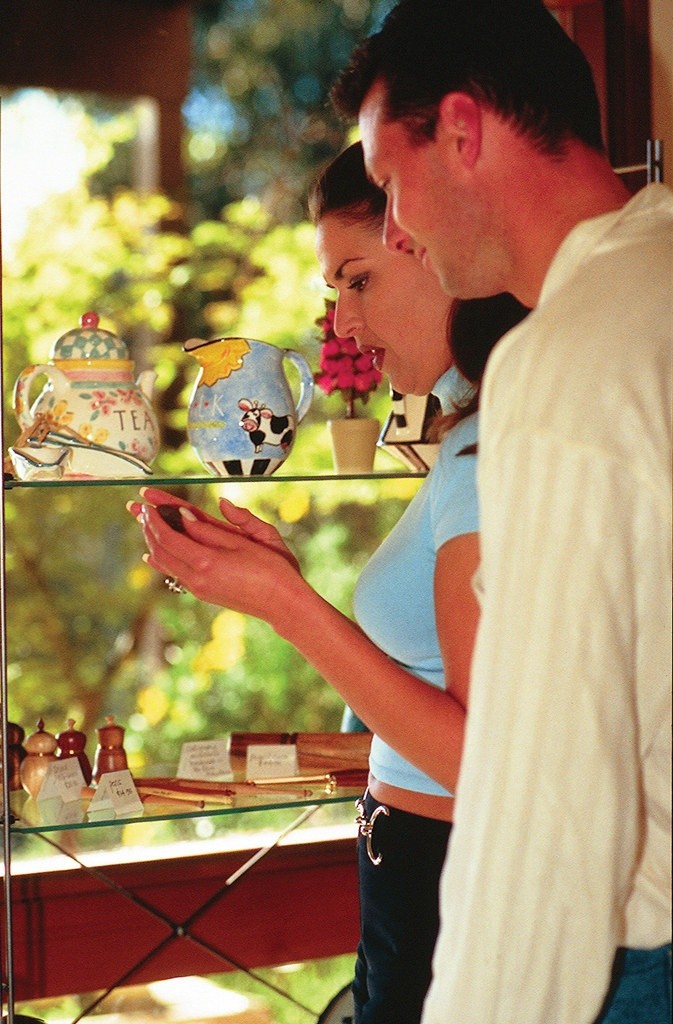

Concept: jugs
[[182, 337, 315, 477]]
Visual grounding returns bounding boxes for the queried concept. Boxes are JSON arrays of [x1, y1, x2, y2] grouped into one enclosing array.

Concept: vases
[[329, 420, 380, 472]]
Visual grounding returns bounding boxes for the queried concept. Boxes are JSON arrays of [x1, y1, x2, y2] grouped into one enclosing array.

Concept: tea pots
[[12, 312, 162, 479]]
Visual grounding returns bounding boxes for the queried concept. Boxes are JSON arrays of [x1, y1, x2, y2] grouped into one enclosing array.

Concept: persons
[[128, 140, 533, 1024], [332, 0, 673, 1024]]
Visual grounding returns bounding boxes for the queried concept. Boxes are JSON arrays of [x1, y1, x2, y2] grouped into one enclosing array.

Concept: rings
[[165, 577, 186, 594]]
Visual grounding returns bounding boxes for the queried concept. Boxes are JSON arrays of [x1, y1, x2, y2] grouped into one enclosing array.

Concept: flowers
[[309, 297, 383, 419]]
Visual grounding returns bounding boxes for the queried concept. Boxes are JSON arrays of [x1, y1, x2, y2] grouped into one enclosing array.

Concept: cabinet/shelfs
[[0, 443, 431, 1024]]
